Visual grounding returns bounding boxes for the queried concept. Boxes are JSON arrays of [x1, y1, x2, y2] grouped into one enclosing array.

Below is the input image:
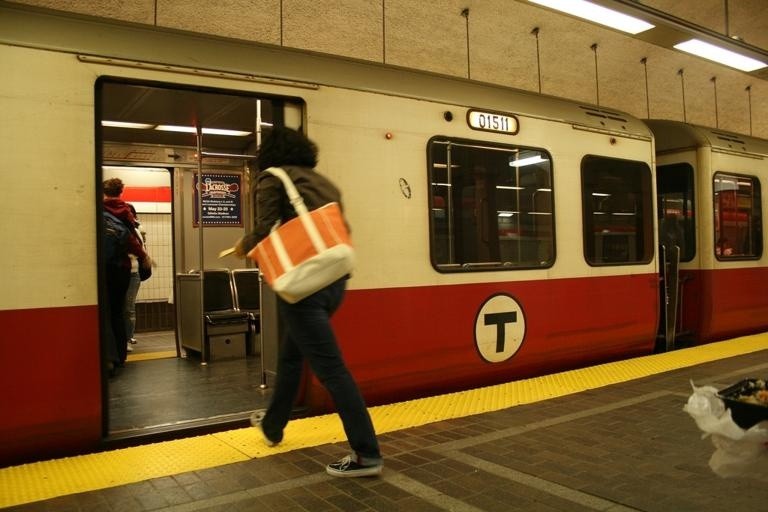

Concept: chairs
[[189, 266, 261, 361]]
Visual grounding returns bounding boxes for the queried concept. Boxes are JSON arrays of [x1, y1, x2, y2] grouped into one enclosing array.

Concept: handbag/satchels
[[136, 256, 152, 281], [246, 202, 356, 304]]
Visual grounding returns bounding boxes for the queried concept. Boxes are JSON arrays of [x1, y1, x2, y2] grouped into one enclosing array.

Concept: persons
[[235, 124, 385, 478], [104, 177, 152, 377]]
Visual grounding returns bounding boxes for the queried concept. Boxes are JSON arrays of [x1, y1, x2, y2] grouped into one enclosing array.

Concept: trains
[[0, 0, 768, 471]]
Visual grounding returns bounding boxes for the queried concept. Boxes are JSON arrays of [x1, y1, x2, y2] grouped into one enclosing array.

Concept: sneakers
[[126, 338, 138, 352], [325, 453, 382, 478], [250, 411, 280, 447]]
